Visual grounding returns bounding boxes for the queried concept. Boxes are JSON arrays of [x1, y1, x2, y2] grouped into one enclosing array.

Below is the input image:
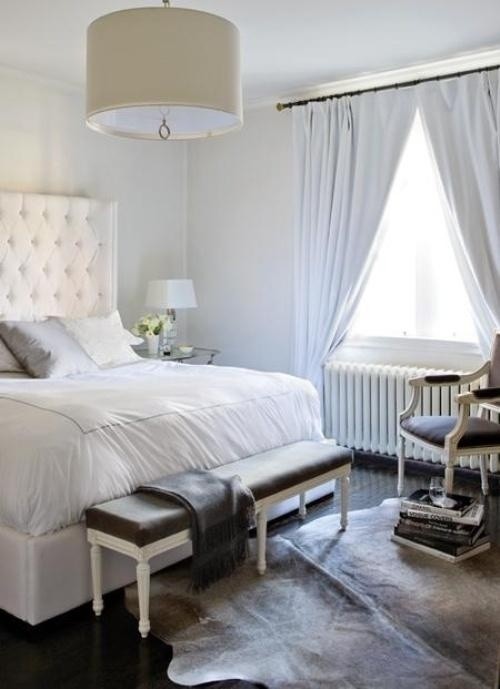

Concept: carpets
[[121, 496, 500, 689]]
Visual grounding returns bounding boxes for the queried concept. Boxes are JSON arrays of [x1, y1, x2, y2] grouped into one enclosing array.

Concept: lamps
[[144, 279, 197, 346], [85, 1, 244, 142]]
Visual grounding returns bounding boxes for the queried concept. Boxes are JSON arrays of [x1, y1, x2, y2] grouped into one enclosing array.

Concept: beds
[[1, 187, 335, 626]]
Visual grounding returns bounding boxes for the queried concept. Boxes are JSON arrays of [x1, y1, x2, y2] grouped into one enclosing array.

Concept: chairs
[[393, 332, 500, 496]]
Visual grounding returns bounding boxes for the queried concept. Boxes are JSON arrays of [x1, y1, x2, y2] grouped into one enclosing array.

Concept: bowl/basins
[[178, 345, 194, 354]]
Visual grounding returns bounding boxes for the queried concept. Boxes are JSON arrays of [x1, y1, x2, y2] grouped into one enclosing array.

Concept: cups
[[429, 477, 447, 504]]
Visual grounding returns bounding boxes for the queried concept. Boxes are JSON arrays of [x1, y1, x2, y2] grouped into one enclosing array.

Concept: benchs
[[84, 440, 354, 639]]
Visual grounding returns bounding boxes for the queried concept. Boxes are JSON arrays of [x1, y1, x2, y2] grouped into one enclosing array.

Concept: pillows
[[0, 309, 144, 379]]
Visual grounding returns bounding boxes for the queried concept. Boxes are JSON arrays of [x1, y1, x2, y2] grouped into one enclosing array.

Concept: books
[[389, 488, 491, 564]]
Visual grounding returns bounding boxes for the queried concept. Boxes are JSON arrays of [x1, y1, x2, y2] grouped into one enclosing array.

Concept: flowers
[[131, 312, 171, 337]]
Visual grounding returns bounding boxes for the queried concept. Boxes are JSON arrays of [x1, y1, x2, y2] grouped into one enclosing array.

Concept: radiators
[[322, 361, 500, 473]]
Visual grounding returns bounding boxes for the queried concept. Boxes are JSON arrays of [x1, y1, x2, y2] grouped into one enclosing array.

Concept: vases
[[146, 335, 159, 355]]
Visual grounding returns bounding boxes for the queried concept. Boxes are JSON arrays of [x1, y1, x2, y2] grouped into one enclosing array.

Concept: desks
[[135, 348, 222, 365]]
